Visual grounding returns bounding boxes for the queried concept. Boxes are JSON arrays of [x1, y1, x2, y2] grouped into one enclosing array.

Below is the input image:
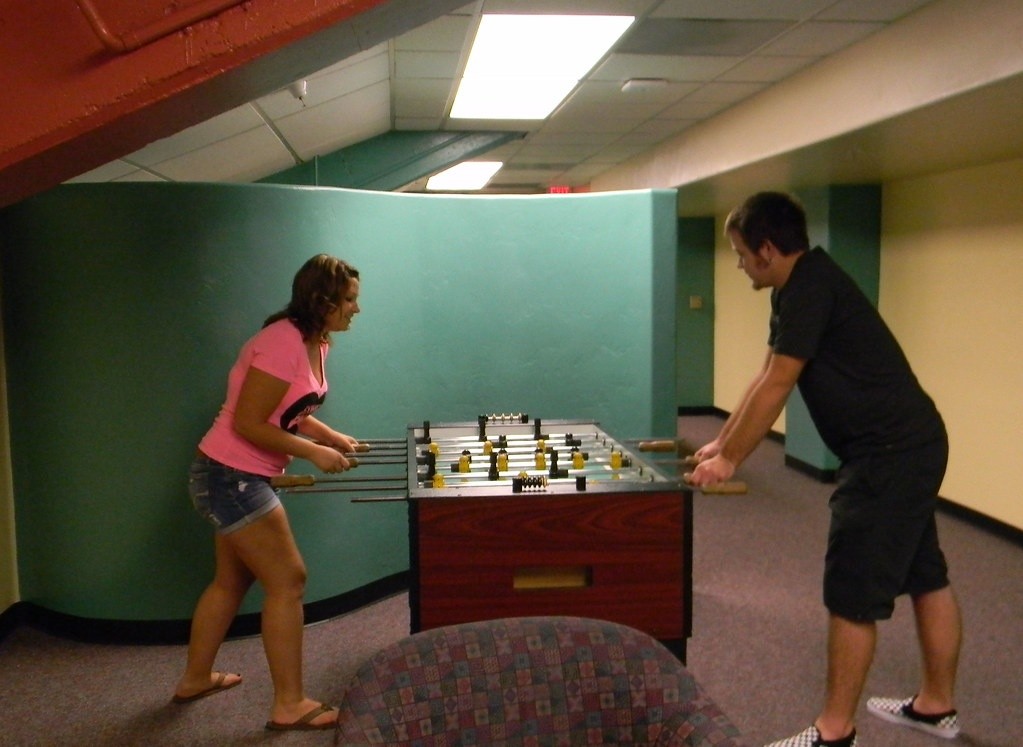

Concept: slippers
[[266, 703, 337, 732], [173, 670, 242, 703]]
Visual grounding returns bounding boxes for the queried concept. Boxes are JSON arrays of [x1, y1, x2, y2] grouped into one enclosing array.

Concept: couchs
[[335, 616, 743, 747]]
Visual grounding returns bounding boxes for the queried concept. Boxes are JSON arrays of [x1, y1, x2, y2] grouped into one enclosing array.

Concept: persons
[[173, 252, 360, 730], [686, 191, 959, 747]]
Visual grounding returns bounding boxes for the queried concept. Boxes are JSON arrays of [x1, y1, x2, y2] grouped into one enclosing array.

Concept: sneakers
[[867, 693, 959, 739], [763, 723, 858, 747]]
